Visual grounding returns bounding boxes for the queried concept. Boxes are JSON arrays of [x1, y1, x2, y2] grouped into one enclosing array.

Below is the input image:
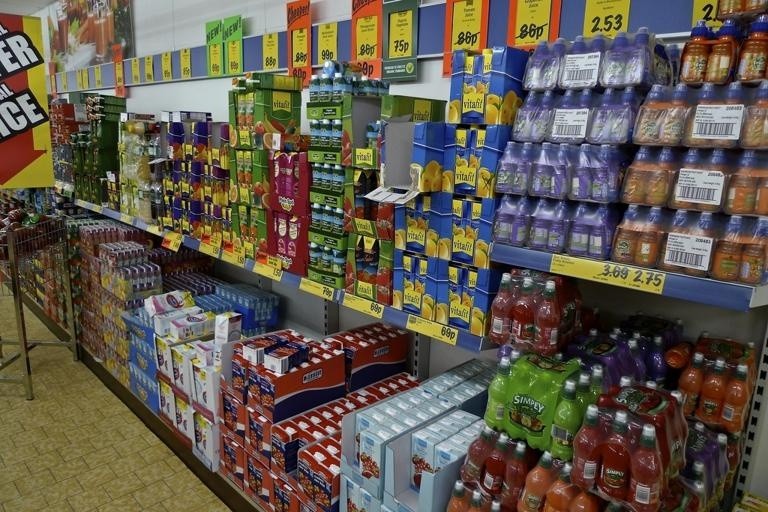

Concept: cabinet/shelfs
[[0, 184, 768, 512]]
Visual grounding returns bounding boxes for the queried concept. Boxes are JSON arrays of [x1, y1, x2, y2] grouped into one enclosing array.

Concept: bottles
[[495, 17, 768, 283], [448, 270, 756, 512]]
